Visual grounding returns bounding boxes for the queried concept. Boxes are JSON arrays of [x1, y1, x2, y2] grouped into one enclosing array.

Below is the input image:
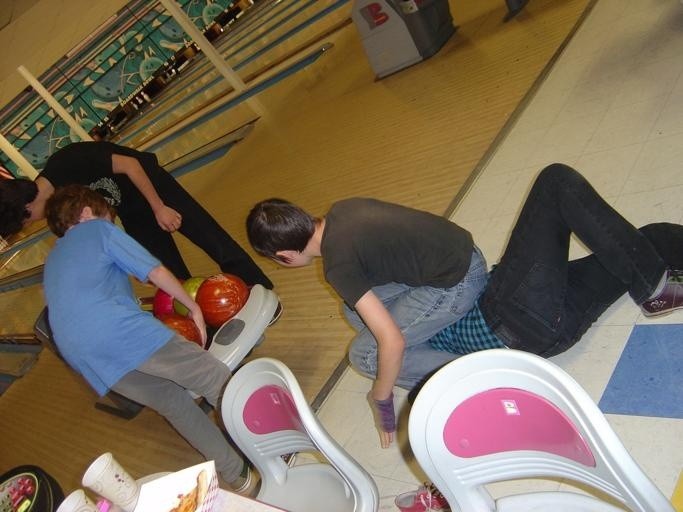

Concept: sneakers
[[395, 484, 451, 511], [230, 460, 254, 495], [642, 271, 683, 317]]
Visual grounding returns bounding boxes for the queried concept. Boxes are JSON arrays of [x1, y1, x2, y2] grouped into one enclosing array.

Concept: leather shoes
[[503, 1, 524, 20]]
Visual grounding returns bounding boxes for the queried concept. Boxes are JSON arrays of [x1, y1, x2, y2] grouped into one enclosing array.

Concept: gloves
[[366, 395, 398, 433]]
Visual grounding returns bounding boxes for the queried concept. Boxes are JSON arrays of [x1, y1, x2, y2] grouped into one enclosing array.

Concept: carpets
[[598, 324, 683, 419]]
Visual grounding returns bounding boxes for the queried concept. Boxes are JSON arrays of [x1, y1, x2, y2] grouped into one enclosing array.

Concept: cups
[[81, 451, 139, 511], [55, 488, 100, 512]]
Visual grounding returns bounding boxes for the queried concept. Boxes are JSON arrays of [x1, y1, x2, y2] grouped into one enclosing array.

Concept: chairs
[[222, 357, 380, 512], [407, 349, 676, 512]]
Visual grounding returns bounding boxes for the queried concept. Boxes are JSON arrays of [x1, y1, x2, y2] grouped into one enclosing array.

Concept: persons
[[0, 141, 283, 327], [45, 185, 252, 493], [245, 198, 487, 448], [430, 163, 683, 359]]
